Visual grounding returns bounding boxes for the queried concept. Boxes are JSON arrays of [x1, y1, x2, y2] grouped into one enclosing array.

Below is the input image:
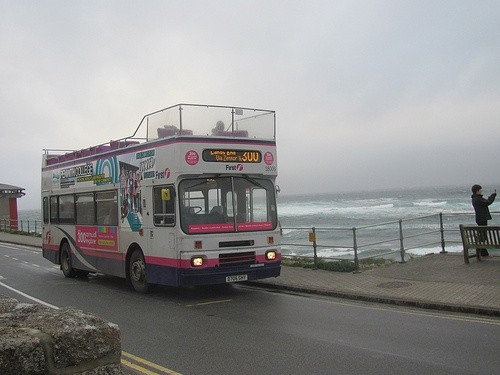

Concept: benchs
[[459, 224, 500, 265]]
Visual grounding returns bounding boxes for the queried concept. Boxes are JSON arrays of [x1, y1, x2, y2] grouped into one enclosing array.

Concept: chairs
[[218, 130, 248, 137], [177, 130, 193, 135], [158, 128, 175, 138], [210, 206, 223, 215], [46, 140, 139, 166], [75, 205, 117, 225]]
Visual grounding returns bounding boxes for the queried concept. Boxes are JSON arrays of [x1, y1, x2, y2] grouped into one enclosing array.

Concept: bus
[[40, 102, 284, 294]]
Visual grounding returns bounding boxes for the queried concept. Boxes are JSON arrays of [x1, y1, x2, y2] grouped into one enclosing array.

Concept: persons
[[471, 185, 497, 257]]
